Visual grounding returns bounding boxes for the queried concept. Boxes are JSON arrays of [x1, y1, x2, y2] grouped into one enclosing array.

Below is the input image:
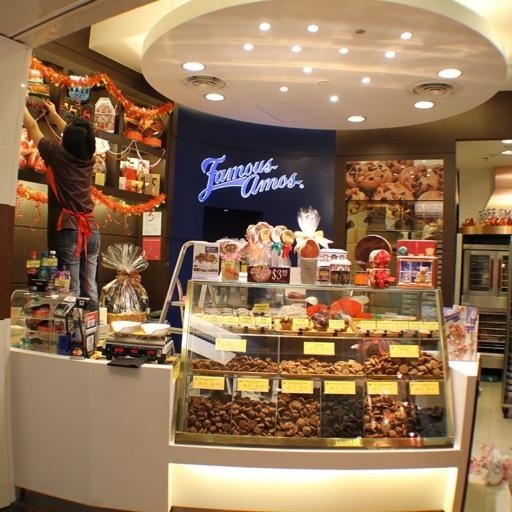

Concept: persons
[[22, 96, 101, 323]]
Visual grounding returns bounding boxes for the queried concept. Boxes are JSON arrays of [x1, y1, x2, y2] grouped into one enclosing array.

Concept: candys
[[218, 220, 296, 267]]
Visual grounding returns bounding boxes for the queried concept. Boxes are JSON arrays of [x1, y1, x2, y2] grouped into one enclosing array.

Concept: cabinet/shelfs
[[17, 45, 176, 204], [173, 285, 456, 449], [10, 289, 90, 359]]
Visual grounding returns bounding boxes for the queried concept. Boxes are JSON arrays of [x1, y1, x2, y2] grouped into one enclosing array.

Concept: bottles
[[26, 251, 41, 291], [47, 250, 59, 290], [477, 208, 512, 227], [58, 265, 71, 293], [38, 252, 50, 291], [128, 160, 145, 176]]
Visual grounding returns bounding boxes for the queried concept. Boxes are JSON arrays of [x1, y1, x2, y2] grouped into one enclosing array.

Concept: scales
[[101, 321, 176, 364]]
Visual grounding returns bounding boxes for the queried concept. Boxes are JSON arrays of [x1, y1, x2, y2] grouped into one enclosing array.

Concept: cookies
[[184, 352, 449, 440], [344, 159, 445, 202]]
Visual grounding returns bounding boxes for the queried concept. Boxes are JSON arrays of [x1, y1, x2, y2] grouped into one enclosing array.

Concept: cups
[[368, 331, 429, 338], [300, 258, 318, 286], [119, 176, 126, 191], [230, 324, 274, 334], [399, 232, 408, 240]]
